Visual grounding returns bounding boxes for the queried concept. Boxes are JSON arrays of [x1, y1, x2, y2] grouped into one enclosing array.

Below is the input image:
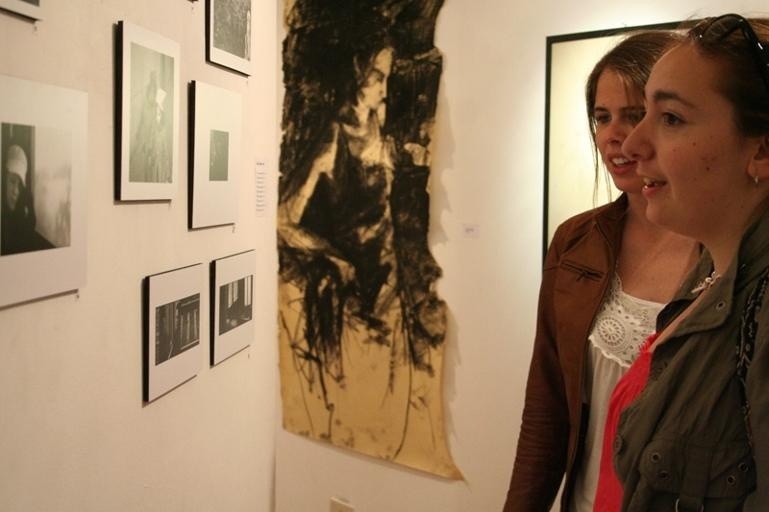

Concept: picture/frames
[[212, 248, 259, 369], [542, 16, 768, 280], [141, 260, 211, 409], [203, 1, 254, 78], [191, 79, 243, 233], [115, 19, 182, 206], [0, 1, 46, 24], [1, 72, 90, 310]]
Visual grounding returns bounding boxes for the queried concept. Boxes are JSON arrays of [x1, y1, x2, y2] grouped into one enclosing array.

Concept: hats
[[6, 145, 27, 188]]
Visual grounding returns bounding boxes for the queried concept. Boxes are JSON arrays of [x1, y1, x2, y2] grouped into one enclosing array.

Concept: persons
[[501, 32, 701, 512], [595, 12, 769, 512], [278, 39, 427, 328], [1, 141, 34, 255]]
